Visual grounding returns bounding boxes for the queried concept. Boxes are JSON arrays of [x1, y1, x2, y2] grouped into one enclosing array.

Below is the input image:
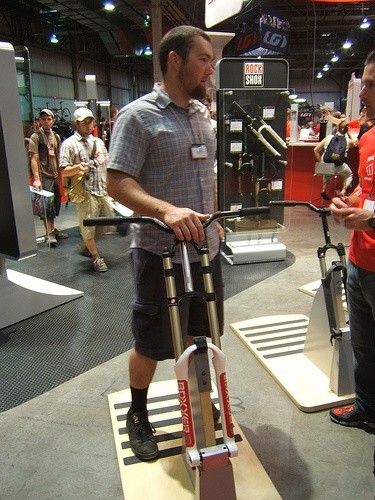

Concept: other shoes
[[321, 192, 331, 200]]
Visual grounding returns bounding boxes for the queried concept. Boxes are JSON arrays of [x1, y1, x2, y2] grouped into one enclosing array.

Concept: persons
[[105, 24, 226, 463], [329, 49, 375, 438], [310, 108, 374, 200], [59, 107, 127, 272], [29, 108, 69, 247]]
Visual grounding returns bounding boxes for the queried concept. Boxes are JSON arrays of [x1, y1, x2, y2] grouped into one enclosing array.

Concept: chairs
[[309, 162, 346, 209]]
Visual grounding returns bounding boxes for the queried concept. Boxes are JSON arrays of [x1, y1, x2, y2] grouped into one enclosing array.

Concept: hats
[[40, 109, 54, 118], [73, 108, 95, 123]]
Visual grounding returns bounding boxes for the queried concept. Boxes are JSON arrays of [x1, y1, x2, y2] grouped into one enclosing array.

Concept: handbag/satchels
[[30, 186, 56, 219], [64, 173, 91, 205]]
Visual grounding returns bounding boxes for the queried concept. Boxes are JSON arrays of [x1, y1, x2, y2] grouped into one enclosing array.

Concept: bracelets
[[369, 211, 375, 229]]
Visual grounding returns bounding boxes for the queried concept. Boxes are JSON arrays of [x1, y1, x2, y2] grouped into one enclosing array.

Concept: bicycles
[[45, 102, 71, 124]]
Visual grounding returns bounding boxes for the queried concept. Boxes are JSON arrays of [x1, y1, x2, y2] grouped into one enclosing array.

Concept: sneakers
[[81, 236, 87, 247], [329, 403, 375, 428], [126, 410, 159, 460], [211, 402, 219, 421], [45, 231, 58, 245], [54, 228, 69, 238], [92, 256, 108, 272]]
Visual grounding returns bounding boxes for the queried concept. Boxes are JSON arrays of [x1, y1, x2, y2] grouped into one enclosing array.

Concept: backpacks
[[323, 134, 348, 166]]
